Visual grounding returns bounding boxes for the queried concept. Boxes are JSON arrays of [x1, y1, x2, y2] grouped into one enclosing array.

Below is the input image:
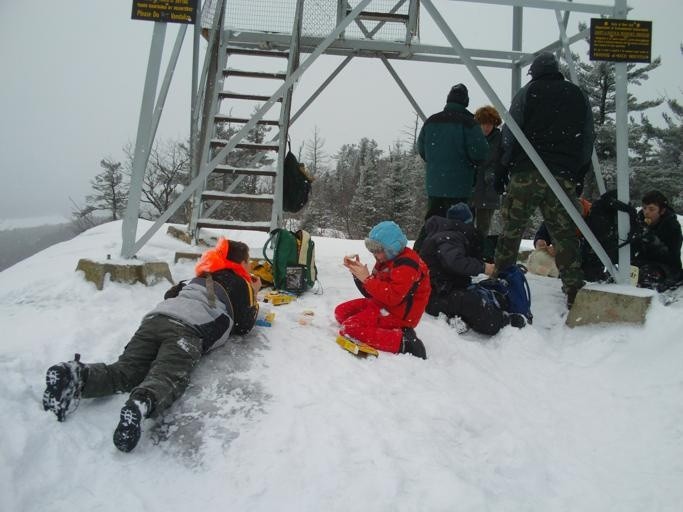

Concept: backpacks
[[280, 144, 312, 214], [497, 260, 534, 324], [262, 229, 318, 295], [587, 189, 638, 250]]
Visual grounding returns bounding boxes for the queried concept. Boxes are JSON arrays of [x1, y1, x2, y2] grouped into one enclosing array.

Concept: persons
[[533, 177, 604, 280], [419, 83, 488, 218], [414, 202, 525, 334], [336, 221, 430, 359], [42, 240, 261, 453], [629, 190, 682, 291], [493, 52, 594, 311], [468, 106, 505, 239]]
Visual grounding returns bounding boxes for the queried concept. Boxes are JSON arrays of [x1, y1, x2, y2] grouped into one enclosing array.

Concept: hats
[[447, 84, 469, 107], [476, 104, 501, 126], [448, 202, 473, 223], [528, 51, 558, 77], [364, 221, 408, 260]]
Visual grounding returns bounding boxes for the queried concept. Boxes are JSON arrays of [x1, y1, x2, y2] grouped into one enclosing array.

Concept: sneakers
[[112, 396, 148, 455], [41, 352, 84, 423], [400, 325, 428, 360], [564, 286, 580, 310]]
